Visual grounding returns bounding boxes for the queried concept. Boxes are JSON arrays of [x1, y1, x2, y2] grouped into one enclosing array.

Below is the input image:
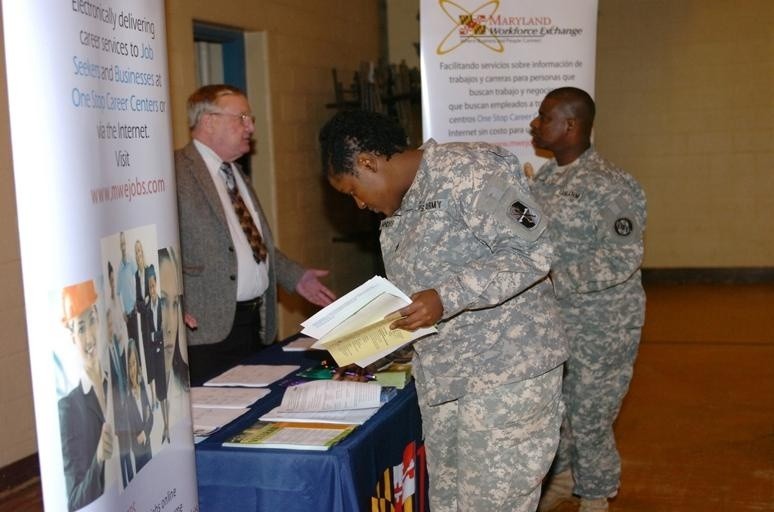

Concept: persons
[[528, 87, 647, 512], [318, 107, 572, 512], [59, 231, 189, 511], [174, 84, 337, 386]]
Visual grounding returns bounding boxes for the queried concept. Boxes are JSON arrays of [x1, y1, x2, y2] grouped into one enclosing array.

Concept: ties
[[219, 162, 268, 265]]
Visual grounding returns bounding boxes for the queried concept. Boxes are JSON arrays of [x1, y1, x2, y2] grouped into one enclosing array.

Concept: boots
[[536, 468, 609, 512]]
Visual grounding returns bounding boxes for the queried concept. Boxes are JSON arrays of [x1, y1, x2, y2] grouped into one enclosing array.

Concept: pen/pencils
[[329, 370, 376, 379]]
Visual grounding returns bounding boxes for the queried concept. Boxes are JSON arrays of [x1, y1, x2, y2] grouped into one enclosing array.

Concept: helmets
[[61, 280, 99, 326]]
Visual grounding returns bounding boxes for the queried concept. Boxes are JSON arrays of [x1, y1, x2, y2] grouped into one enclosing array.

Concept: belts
[[237, 297, 263, 313]]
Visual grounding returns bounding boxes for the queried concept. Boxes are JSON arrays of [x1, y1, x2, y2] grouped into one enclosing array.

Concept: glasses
[[210, 112, 256, 126]]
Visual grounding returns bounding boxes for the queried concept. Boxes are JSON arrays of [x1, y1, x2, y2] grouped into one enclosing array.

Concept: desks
[[190, 328, 430, 512]]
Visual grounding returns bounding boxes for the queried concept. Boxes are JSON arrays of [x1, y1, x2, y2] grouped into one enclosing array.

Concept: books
[[190, 361, 412, 451], [299, 276, 438, 368], [282, 337, 316, 352]]
